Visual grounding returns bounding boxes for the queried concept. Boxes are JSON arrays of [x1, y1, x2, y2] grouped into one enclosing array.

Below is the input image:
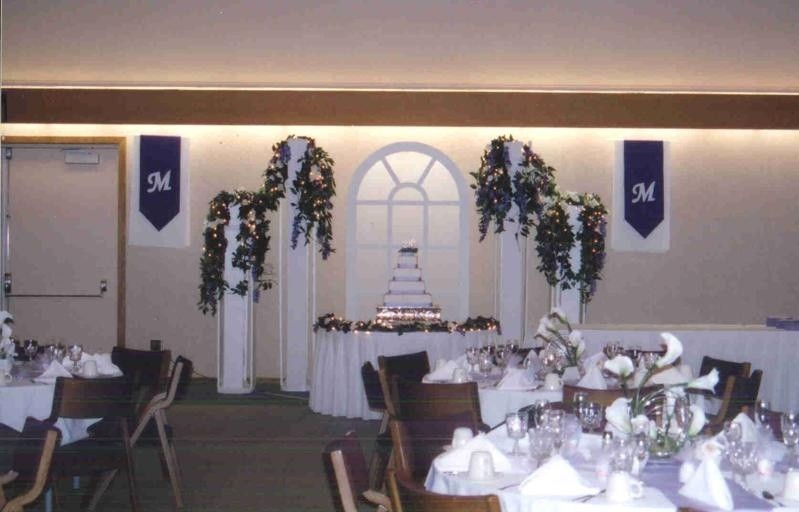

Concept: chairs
[[0, 339, 193, 512]]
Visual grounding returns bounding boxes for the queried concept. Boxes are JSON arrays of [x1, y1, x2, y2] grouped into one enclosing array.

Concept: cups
[[433, 358, 799, 506], [83, 360, 99, 377], [0, 367, 13, 386]]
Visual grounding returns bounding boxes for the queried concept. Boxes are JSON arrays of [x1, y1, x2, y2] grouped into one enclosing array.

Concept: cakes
[[383, 239, 432, 308]]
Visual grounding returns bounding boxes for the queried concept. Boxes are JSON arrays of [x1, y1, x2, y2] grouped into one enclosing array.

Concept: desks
[[307, 326, 500, 422]]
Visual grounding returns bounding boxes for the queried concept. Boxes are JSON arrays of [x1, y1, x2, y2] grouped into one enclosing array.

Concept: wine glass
[[21, 339, 83, 372], [468, 341, 799, 484]]
